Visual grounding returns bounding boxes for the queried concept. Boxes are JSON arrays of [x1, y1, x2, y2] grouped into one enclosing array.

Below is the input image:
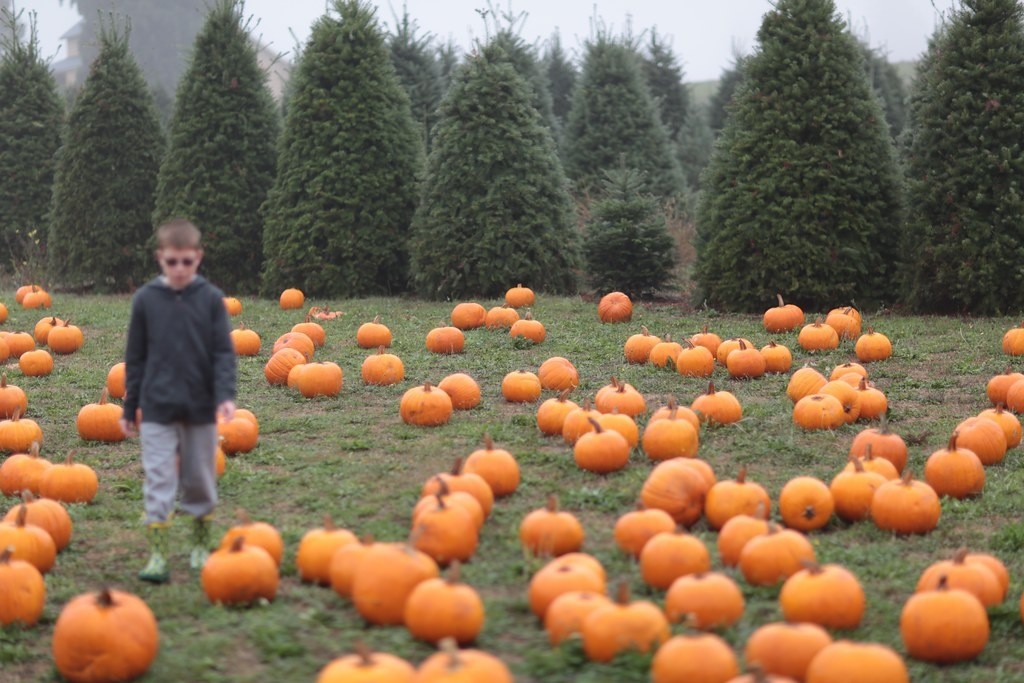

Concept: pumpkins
[[0, 284, 1024, 683]]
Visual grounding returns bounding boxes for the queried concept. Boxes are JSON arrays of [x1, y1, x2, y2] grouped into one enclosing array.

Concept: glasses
[[161, 255, 201, 267]]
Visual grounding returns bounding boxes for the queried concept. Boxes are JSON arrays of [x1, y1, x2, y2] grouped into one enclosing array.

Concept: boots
[[191, 512, 212, 572], [136, 518, 174, 583]]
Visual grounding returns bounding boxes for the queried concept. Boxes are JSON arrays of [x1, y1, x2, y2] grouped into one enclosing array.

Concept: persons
[[120, 227, 238, 583]]
